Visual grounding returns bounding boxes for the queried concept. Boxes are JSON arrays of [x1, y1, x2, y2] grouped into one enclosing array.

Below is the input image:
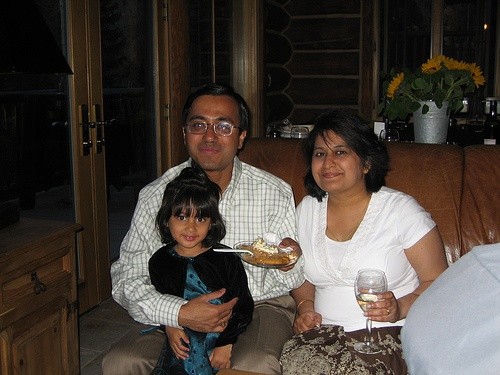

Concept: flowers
[[385, 54, 484, 118]]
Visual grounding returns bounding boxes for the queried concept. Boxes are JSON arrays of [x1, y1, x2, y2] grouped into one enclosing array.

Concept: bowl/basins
[[279, 124, 315, 138]]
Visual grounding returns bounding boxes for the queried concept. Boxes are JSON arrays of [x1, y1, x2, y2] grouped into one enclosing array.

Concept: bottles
[[482, 100, 500, 146], [269, 123, 280, 138]]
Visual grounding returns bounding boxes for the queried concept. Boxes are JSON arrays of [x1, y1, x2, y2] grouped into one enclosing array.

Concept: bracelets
[[296, 298, 314, 310]]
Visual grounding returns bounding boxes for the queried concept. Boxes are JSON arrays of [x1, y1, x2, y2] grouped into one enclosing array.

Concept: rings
[[387, 308, 390, 315], [221, 319, 225, 327]]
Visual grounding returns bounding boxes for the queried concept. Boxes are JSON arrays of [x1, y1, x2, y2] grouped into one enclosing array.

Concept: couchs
[[235, 136, 500, 267]]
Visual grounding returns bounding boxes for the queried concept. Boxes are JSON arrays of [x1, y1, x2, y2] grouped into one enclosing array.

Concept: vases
[[413, 99, 450, 145]]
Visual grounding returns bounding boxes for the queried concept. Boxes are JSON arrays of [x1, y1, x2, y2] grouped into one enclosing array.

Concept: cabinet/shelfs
[[0, 214, 85, 375]]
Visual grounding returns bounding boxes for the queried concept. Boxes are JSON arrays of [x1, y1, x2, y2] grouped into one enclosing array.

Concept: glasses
[[186, 121, 239, 137]]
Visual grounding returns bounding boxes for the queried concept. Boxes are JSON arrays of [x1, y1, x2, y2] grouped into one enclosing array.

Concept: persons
[[101, 84, 305, 375], [279, 107, 448, 375], [149, 167, 254, 375]]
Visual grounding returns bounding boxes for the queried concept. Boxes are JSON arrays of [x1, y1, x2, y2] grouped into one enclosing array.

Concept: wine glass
[[354, 268, 388, 355]]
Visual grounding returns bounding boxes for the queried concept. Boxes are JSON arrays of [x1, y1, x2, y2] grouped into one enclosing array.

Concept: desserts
[[238, 236, 296, 266]]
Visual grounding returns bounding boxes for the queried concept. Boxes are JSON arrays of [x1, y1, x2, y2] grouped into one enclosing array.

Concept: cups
[[291, 127, 309, 138], [379, 129, 399, 143]]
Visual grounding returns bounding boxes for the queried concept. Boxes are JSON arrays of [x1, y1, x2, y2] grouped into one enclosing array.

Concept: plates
[[233, 240, 299, 269]]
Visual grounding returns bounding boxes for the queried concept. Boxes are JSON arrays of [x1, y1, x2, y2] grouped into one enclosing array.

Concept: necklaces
[[327, 209, 366, 242]]
